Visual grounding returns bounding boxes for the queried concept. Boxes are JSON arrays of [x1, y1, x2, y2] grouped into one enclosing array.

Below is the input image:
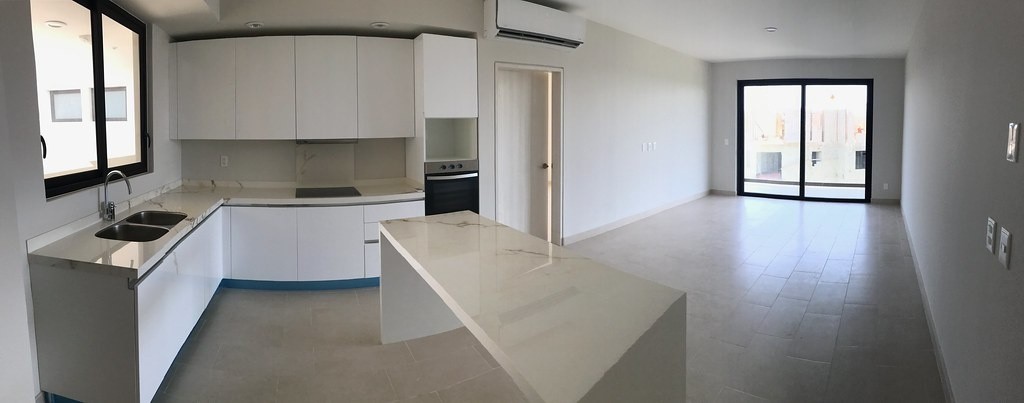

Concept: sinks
[[94, 222, 170, 243], [126, 210, 188, 226]]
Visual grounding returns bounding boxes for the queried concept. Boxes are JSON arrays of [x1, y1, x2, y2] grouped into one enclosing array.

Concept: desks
[[377, 209, 688, 403]]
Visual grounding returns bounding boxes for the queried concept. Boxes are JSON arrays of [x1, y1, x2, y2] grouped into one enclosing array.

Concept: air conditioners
[[484, 0, 585, 53]]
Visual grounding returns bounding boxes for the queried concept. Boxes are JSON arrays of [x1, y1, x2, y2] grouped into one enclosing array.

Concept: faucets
[[104, 170, 132, 217]]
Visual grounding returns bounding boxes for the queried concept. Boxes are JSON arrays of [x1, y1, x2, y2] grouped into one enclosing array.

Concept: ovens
[[425, 160, 479, 216]]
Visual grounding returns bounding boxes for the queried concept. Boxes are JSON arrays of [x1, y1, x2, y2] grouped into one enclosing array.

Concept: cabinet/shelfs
[[25, 200, 426, 403], [168, 35, 417, 140]]
